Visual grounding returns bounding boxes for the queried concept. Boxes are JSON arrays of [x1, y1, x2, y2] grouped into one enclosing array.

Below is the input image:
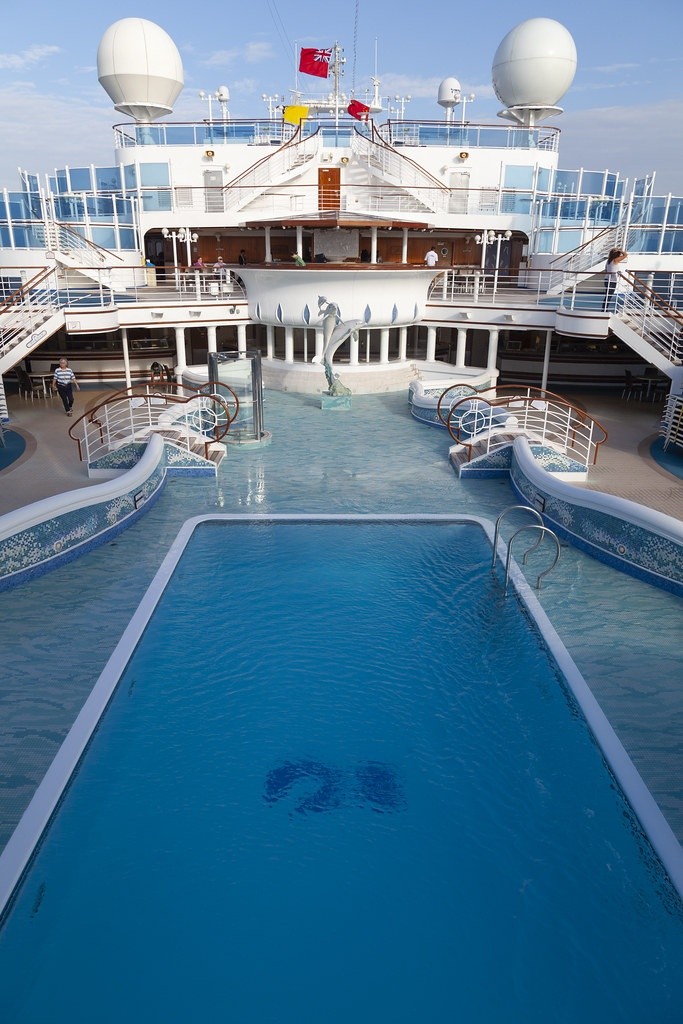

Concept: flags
[[347, 100, 370, 122], [299, 47, 332, 78], [283, 105, 309, 126]]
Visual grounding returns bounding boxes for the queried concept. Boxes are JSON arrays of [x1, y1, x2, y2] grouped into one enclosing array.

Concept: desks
[[26, 371, 59, 400], [635, 374, 667, 401]]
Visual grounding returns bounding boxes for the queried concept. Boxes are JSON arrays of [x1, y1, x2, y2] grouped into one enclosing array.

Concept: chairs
[[652, 379, 671, 404], [640, 367, 658, 397], [446, 263, 485, 295], [620, 370, 643, 401], [18, 370, 45, 403], [49, 363, 61, 399], [163, 364, 178, 395], [13, 366, 33, 398]]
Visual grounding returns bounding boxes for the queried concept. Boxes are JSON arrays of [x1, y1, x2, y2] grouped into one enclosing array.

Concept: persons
[[151, 362, 165, 392], [52, 358, 80, 416], [424, 246, 438, 267], [236, 249, 246, 285], [290, 251, 306, 266], [195, 256, 207, 288], [601, 249, 628, 312], [213, 256, 227, 286]]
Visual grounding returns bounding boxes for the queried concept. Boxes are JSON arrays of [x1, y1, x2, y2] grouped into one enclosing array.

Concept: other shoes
[[70, 407, 73, 412], [66, 410, 72, 418]]
